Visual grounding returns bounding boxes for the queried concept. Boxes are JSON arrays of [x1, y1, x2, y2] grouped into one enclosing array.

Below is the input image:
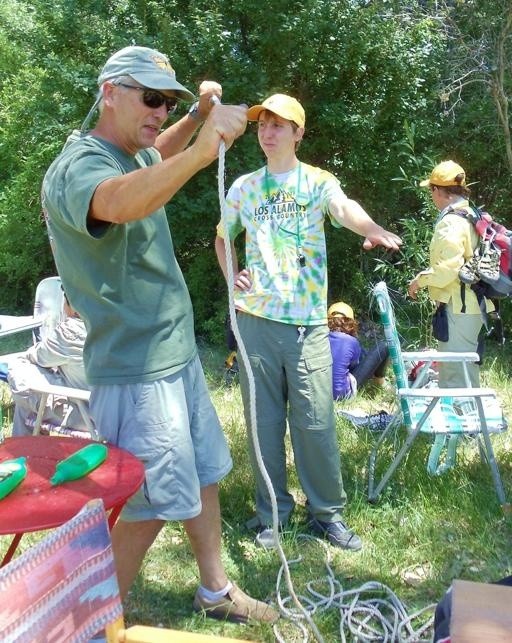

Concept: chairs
[[1, 495, 266, 643], [365, 280, 507, 510], [24, 382, 98, 440], [1, 275, 67, 382]]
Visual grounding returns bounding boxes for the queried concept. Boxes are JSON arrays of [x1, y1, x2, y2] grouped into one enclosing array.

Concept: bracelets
[[189, 101, 206, 125]]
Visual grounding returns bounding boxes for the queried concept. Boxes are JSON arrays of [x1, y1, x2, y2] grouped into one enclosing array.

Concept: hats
[[96, 45, 196, 104], [419, 160, 466, 190], [329, 301, 355, 320], [245, 93, 306, 133]]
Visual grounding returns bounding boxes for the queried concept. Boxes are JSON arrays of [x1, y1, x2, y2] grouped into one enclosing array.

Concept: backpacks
[[438, 203, 511, 300]]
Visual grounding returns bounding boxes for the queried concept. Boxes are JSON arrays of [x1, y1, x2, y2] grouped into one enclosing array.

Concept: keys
[[296, 326, 308, 345]]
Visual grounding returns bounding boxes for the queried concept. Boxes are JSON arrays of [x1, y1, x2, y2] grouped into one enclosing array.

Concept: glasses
[[110, 81, 180, 114]]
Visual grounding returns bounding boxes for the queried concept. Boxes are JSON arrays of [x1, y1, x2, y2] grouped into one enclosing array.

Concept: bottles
[[50, 440, 111, 484], [1, 456, 29, 500]]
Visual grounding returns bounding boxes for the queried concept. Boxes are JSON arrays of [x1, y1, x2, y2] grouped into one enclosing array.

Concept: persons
[[409, 160, 494, 441], [6, 271, 89, 438], [215, 93, 404, 551], [42, 44, 280, 629], [324, 301, 390, 403]]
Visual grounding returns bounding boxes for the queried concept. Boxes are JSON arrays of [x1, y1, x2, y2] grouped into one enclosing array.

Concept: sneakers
[[192, 585, 281, 626], [305, 513, 362, 553], [256, 525, 283, 549]]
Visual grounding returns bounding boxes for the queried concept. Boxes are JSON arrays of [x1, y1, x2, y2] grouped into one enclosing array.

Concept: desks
[[1, 435, 146, 573], [1, 313, 44, 338]]
